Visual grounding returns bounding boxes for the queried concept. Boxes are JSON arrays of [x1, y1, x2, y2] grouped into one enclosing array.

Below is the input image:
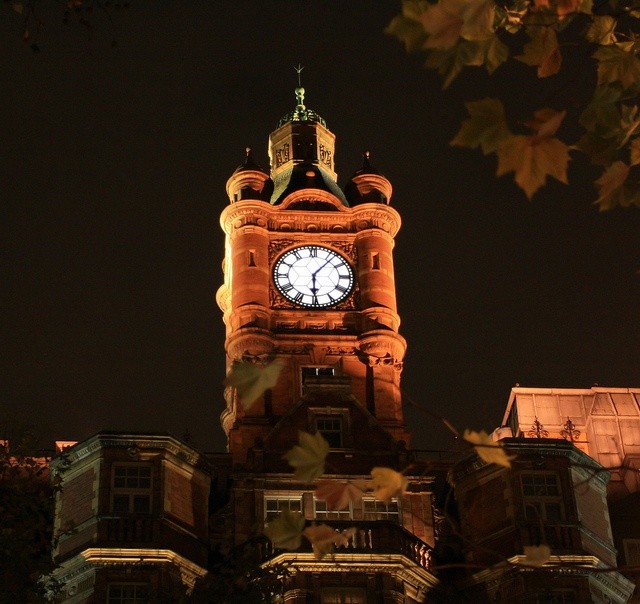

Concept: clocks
[[272, 243, 356, 310]]
